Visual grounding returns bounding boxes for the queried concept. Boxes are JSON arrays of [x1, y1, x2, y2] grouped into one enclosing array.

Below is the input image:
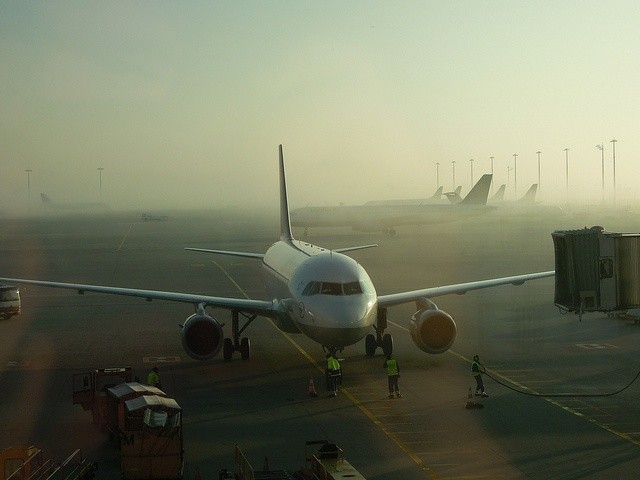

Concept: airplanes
[[289, 174, 538, 237], [1, 143, 555, 391]]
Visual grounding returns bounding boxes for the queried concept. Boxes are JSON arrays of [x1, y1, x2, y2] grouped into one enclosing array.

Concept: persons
[[471, 354, 489, 397], [146, 367, 160, 387], [382, 356, 403, 399], [326, 353, 342, 398]]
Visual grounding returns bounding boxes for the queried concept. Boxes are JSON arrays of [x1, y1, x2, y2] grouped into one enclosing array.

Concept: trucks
[[74, 367, 133, 429], [0, 284, 22, 321]]
